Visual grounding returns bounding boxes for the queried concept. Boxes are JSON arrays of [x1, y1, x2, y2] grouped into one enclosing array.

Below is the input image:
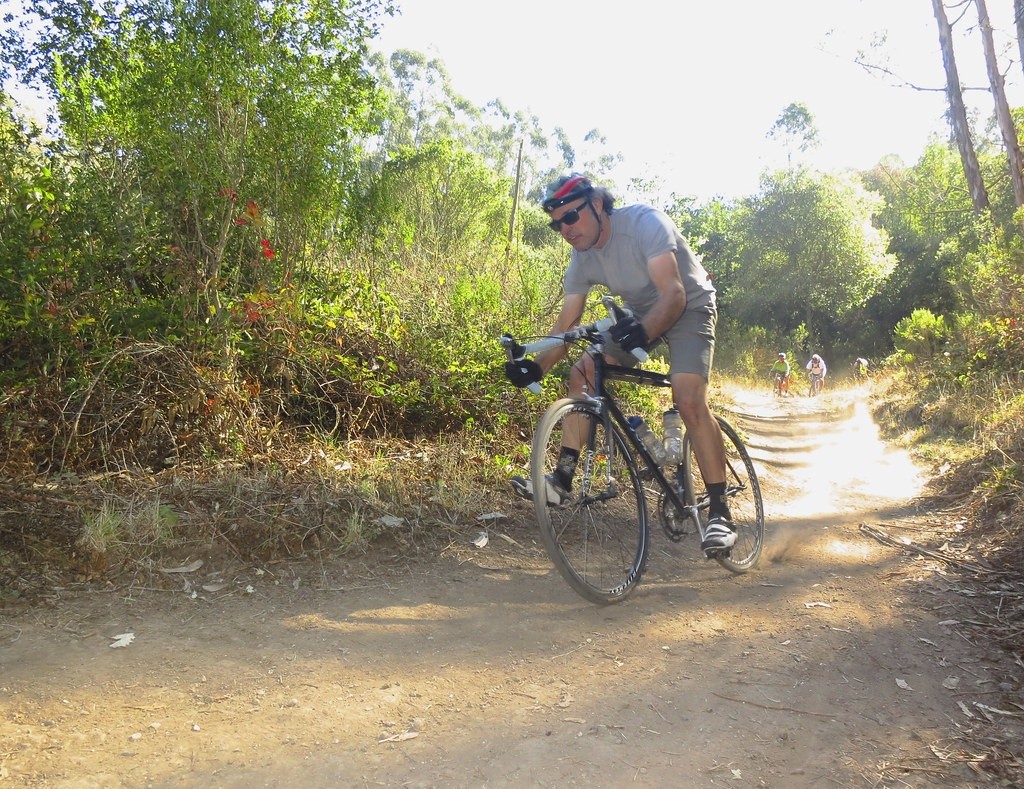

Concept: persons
[[769, 353, 790, 397], [805, 354, 825, 394], [504, 172, 738, 555], [854, 357, 868, 377]]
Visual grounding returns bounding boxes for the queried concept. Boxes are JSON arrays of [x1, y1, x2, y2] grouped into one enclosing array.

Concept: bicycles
[[806, 373, 824, 398], [500, 295, 764, 605], [771, 371, 789, 399]]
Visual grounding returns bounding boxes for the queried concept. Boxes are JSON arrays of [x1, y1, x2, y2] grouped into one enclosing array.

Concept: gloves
[[506, 359, 543, 389], [609, 317, 649, 352]]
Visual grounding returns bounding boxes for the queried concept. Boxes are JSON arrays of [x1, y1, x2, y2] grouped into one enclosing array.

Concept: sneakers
[[509, 475, 571, 511], [701, 513, 740, 554]]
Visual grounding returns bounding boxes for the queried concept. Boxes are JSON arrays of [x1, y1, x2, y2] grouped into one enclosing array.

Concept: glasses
[[546, 200, 590, 233]]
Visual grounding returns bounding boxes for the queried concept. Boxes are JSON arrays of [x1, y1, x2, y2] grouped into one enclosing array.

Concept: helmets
[[542, 171, 594, 212], [812, 354, 820, 360], [778, 353, 785, 358], [856, 358, 861, 362]]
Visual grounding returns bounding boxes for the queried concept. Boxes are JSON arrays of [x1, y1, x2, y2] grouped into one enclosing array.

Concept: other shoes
[[773, 389, 777, 392], [783, 394, 788, 397]]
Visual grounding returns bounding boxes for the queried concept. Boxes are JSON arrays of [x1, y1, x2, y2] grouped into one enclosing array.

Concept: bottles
[[629, 415, 667, 467], [662, 408, 681, 465]]
